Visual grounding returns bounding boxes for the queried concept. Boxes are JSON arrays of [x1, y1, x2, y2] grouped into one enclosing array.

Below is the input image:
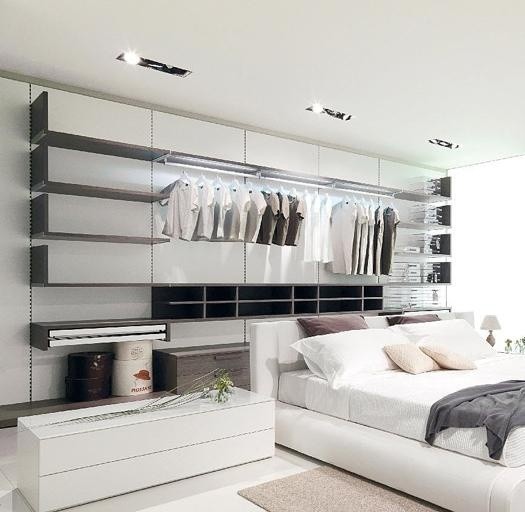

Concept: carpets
[[238, 465, 450, 512]]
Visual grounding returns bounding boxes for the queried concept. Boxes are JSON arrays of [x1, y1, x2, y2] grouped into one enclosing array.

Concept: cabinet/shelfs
[[378, 175, 453, 286], [152, 283, 384, 324], [152, 342, 251, 395], [29, 90, 170, 286]]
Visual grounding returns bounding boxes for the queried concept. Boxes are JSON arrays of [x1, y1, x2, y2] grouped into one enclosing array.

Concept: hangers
[[170, 166, 398, 214]]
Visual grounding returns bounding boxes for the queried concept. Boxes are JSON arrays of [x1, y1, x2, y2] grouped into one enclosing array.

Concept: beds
[[249, 311, 525, 512]]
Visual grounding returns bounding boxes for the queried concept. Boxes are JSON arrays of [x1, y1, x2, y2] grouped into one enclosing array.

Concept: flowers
[[7, 367, 236, 435]]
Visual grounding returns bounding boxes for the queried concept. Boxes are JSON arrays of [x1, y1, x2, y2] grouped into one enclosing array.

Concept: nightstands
[[15, 385, 276, 512]]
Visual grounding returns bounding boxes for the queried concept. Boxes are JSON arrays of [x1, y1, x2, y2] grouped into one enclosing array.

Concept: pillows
[[289, 314, 500, 393]]
